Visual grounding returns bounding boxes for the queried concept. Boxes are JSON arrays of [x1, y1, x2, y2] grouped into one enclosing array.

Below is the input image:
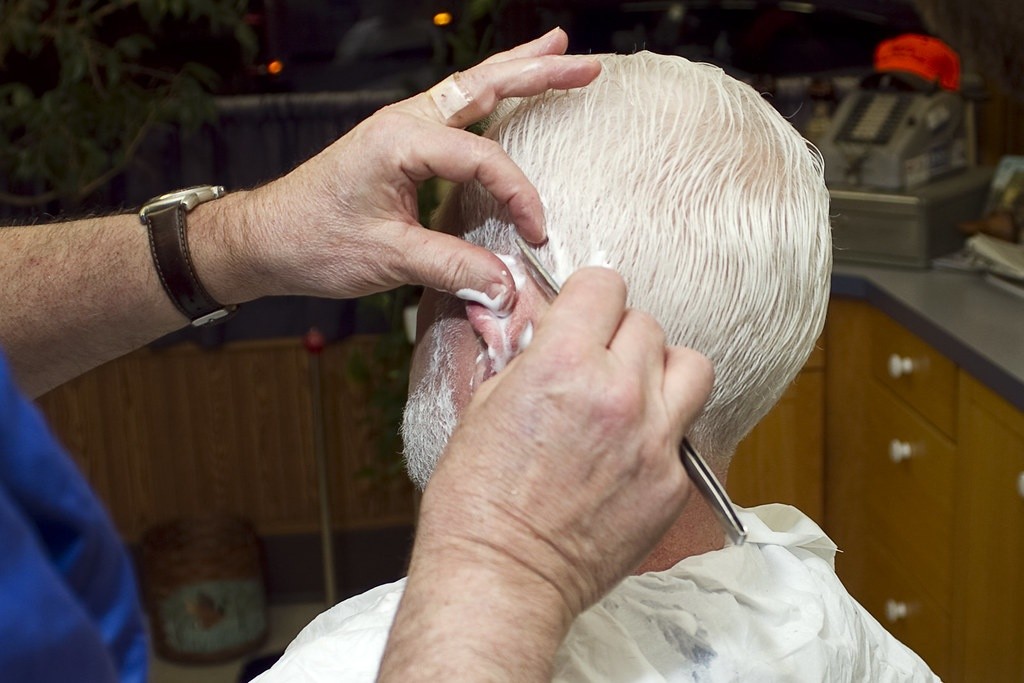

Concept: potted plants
[[341, 1, 540, 488], [0, 0, 258, 216]]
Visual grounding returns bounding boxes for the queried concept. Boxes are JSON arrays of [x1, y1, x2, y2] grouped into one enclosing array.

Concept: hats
[[875, 35, 961, 92]]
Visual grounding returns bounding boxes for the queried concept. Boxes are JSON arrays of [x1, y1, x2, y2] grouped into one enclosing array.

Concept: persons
[[0, 26, 715, 683], [247, 50, 940, 683]]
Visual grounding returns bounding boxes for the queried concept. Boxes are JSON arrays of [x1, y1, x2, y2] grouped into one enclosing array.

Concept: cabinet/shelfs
[[33, 261, 1024, 683]]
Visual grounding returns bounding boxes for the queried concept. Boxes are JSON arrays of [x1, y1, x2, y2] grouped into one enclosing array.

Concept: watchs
[[141, 184, 239, 326]]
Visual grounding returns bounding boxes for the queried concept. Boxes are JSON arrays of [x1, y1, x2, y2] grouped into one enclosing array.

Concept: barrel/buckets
[[142, 515, 268, 664]]
